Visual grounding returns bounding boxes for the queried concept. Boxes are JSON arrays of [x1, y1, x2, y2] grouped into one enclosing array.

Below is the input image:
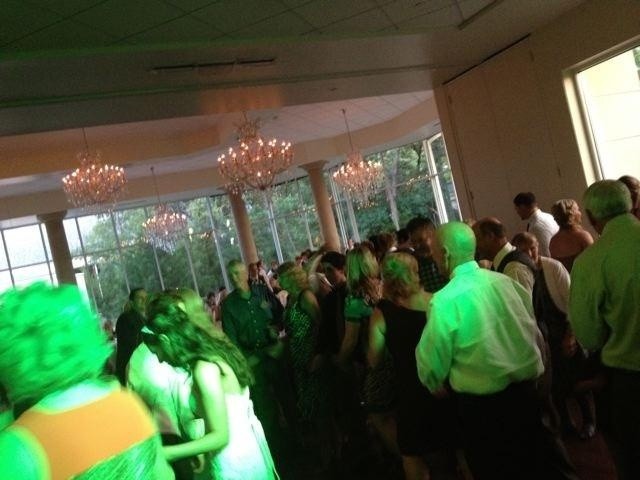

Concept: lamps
[[61, 149, 129, 223], [214, 118, 296, 202], [139, 209, 191, 258], [331, 151, 384, 210]]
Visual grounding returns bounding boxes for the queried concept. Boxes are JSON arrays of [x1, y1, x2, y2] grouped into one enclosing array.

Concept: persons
[[206, 258, 327, 476], [548, 199, 593, 273], [513, 191, 560, 259], [414, 222, 574, 480], [567, 180, 640, 480], [117, 288, 281, 480], [474, 217, 594, 466], [278, 215, 463, 480], [1, 280, 177, 480], [619, 174, 640, 218]]
